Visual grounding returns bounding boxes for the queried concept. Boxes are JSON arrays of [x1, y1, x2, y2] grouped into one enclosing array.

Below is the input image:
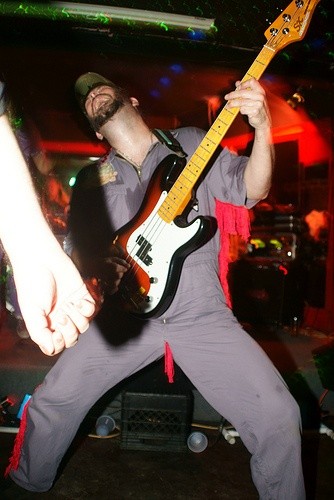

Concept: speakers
[[119, 382, 195, 454]]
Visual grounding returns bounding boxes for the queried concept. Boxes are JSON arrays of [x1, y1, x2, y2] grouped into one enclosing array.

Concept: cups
[[94, 416, 116, 436], [188, 431, 208, 453]]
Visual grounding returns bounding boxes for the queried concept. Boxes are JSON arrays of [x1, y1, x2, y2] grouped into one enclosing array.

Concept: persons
[[3, 70, 309, 500], [1, 59, 100, 362]]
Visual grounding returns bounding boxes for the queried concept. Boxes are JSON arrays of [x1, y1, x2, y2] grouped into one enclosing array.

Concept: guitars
[[93, 0, 319, 320]]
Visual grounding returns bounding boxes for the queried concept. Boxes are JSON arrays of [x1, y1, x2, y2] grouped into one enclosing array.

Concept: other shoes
[[0, 473, 36, 496]]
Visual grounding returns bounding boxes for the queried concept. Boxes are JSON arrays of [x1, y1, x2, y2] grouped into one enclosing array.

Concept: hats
[[74, 71, 109, 99]]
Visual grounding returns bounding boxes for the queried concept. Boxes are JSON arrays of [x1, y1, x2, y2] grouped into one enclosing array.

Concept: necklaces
[[116, 130, 155, 181]]
